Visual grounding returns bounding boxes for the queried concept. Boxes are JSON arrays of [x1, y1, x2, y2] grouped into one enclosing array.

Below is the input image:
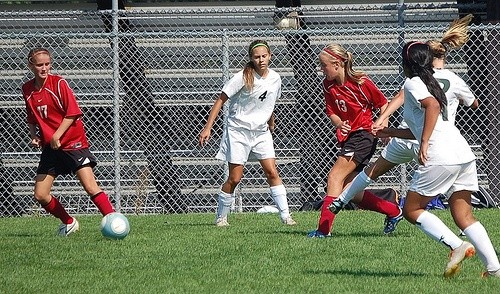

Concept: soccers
[[100, 212, 131, 240]]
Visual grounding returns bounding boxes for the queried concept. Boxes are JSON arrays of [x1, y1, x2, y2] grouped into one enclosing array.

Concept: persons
[[403, 40, 500, 277], [22, 49, 115, 236], [326, 14, 474, 235], [309, 44, 403, 237], [199, 40, 296, 226]]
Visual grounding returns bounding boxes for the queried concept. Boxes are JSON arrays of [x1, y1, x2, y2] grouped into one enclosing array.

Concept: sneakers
[[328, 198, 345, 214], [281, 215, 297, 226], [215, 215, 228, 227], [383, 206, 403, 234], [444, 241, 475, 277], [481, 266, 500, 278], [306, 229, 331, 238], [56, 216, 79, 238]]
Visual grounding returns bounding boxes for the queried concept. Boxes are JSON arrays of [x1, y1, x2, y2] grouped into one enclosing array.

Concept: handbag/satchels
[[469, 187, 495, 209], [426, 197, 446, 210], [367, 189, 400, 204], [300, 202, 322, 212]]
